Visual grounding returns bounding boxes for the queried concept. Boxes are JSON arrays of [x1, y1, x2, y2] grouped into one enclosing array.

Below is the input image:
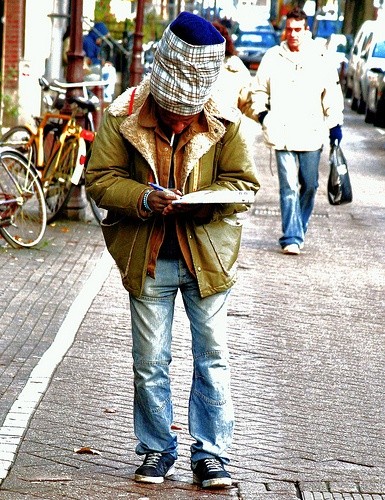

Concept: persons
[[251, 8, 345, 254], [203, 22, 254, 221], [83, 22, 107, 65], [85, 11, 259, 464]]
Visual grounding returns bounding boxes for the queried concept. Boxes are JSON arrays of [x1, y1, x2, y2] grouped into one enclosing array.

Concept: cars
[[365, 66, 385, 127], [314, 13, 385, 124], [232, 29, 279, 72]]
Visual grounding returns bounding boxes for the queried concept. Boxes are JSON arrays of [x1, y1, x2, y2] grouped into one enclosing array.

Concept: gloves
[[329, 125, 343, 147], [257, 111, 268, 124]]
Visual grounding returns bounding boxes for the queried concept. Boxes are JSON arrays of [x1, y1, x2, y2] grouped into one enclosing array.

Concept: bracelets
[[144, 189, 155, 212]]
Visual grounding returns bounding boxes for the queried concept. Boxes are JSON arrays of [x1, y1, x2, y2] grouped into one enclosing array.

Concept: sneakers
[[134, 451, 175, 484], [191, 457, 231, 488]]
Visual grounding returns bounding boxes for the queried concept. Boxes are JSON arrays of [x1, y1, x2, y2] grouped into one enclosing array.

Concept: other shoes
[[283, 242, 304, 255]]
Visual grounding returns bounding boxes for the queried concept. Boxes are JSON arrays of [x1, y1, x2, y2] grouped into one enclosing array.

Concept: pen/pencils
[[148, 181, 181, 199]]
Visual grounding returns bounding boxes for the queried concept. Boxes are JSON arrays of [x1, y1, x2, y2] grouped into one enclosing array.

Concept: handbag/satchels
[[327, 146, 353, 205]]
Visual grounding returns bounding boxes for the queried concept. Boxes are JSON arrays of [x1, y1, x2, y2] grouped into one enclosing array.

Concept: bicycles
[[1, 140, 48, 249], [1, 77, 95, 224], [24, 79, 105, 226]]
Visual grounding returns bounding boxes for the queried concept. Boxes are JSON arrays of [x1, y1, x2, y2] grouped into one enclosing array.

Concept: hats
[[150, 12, 227, 116]]
